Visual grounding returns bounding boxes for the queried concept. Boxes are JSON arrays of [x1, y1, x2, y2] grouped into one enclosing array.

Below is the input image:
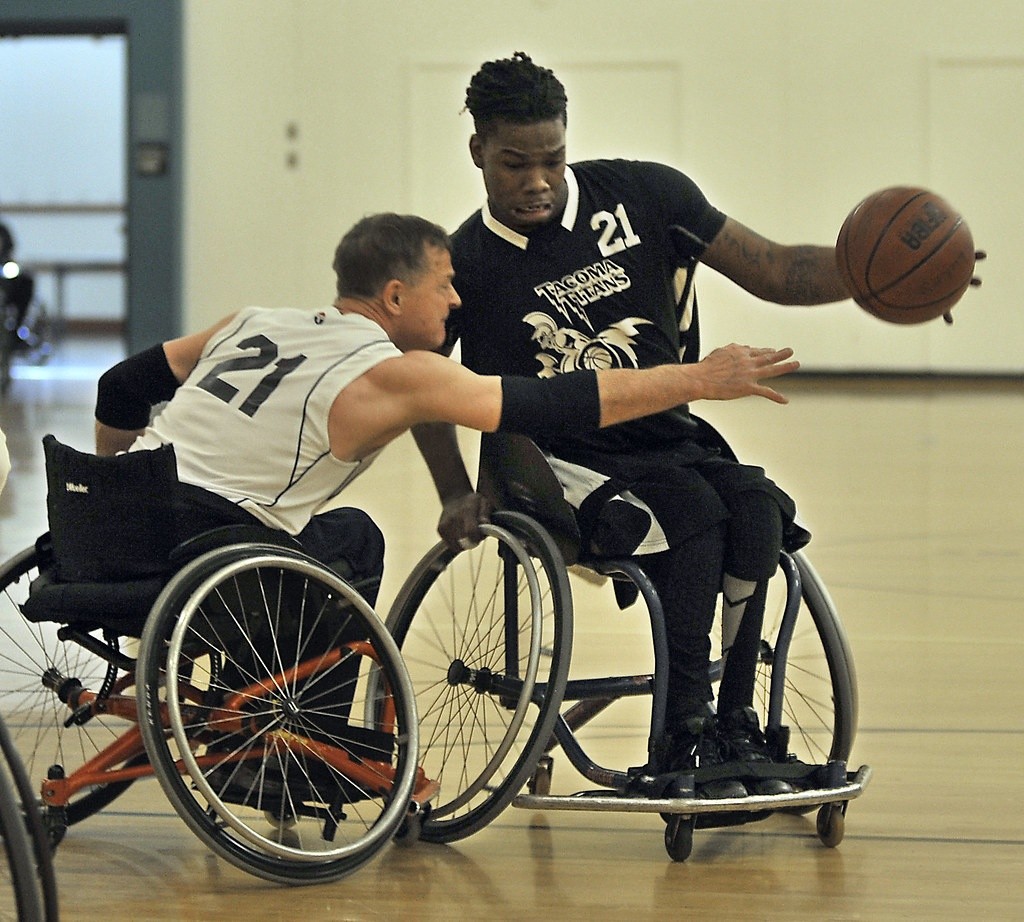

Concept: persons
[[95, 209, 800, 725], [410, 50, 989, 799]]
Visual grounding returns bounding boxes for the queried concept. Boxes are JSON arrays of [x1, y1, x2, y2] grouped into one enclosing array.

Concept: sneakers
[[663, 705, 748, 799], [720, 708, 795, 795]]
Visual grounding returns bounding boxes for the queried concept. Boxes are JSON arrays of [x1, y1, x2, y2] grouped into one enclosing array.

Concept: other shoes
[[201, 733, 360, 804]]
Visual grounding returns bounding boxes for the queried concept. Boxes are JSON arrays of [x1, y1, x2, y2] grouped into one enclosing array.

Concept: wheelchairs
[[0, 431, 437, 884], [362, 409, 875, 863]]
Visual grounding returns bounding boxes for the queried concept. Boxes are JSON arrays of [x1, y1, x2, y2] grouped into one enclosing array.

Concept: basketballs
[[835, 185, 975, 324]]
